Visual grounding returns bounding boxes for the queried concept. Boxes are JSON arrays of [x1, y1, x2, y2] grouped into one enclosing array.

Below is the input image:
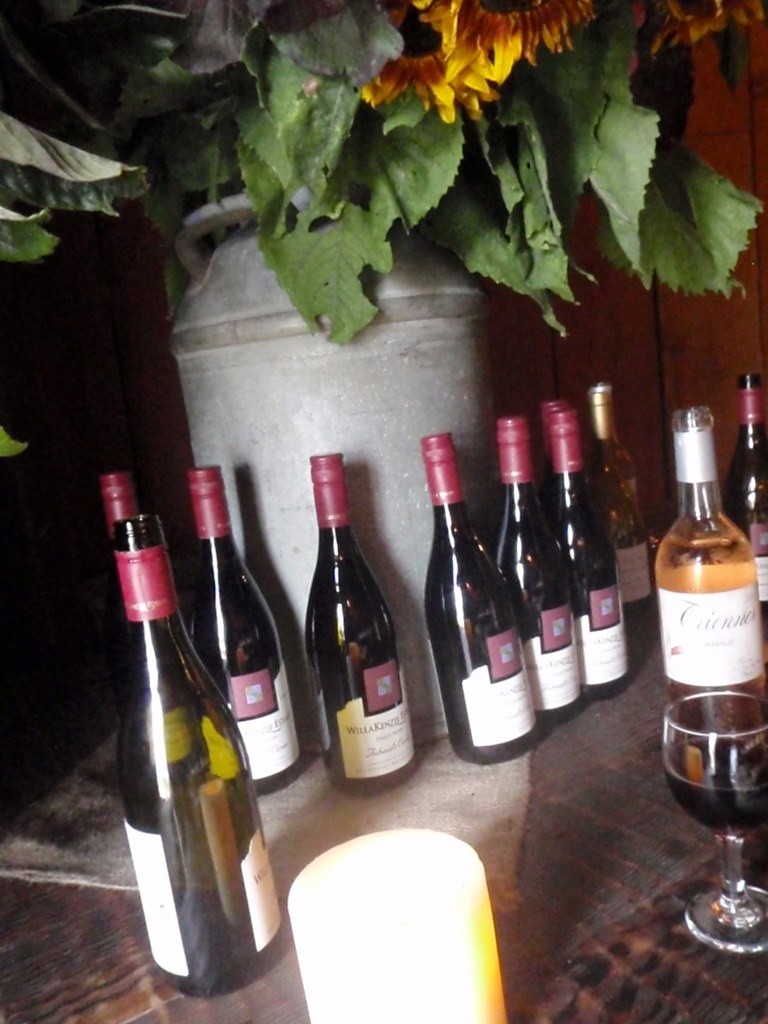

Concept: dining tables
[[1, 529, 768, 1024]]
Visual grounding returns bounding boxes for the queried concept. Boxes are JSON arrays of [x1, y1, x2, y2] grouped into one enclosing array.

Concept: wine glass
[[661, 688, 768, 958]]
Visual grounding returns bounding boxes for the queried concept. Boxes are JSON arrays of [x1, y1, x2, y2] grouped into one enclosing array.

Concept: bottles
[[302, 454, 417, 796], [417, 383, 653, 767], [653, 405, 767, 705], [180, 462, 302, 796], [93, 468, 286, 1000], [724, 376, 767, 609]]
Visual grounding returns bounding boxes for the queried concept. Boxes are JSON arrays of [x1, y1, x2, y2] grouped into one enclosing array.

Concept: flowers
[[259, 2, 768, 128]]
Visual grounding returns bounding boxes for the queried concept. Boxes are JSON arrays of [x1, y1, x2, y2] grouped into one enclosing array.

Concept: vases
[[169, 174, 506, 766]]
